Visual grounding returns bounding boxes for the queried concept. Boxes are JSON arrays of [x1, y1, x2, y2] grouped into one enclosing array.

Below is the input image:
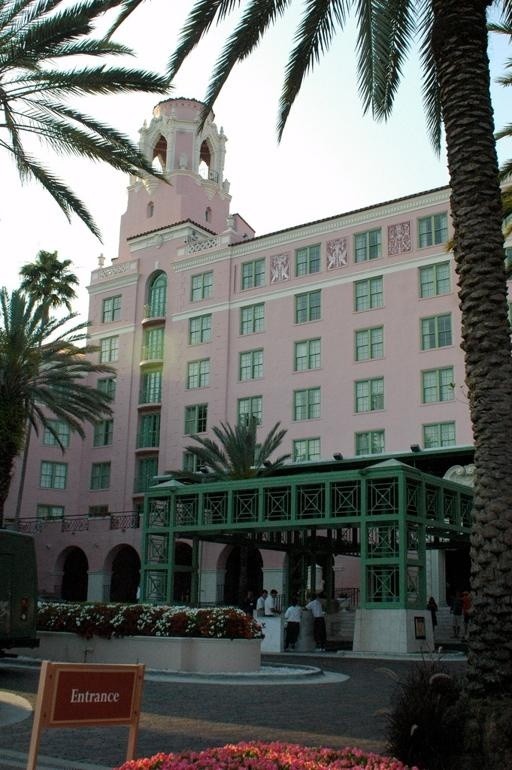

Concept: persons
[[265, 589, 282, 617], [450, 594, 464, 639], [303, 593, 327, 652], [284, 599, 303, 653], [459, 592, 475, 638], [426, 597, 437, 632], [241, 590, 255, 617], [256, 590, 268, 617]]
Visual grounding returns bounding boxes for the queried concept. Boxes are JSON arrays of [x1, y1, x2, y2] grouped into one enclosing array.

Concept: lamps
[[200, 467, 209, 474], [334, 452, 342, 461], [410, 444, 420, 452], [262, 460, 271, 467]]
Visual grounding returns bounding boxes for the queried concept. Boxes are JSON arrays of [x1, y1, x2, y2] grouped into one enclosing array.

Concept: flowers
[[34, 599, 266, 638]]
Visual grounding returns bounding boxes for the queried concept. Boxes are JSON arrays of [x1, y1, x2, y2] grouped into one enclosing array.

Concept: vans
[[0, 529, 40, 657]]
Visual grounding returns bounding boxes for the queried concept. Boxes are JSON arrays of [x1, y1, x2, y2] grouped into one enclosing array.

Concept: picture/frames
[[414, 617, 426, 639]]
